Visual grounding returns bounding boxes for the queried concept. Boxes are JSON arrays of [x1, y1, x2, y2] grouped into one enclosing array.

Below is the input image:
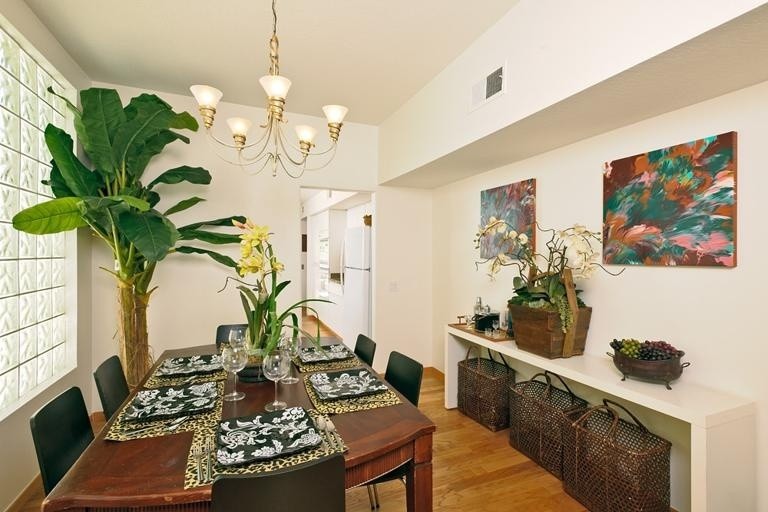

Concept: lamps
[[189, 0, 348, 179]]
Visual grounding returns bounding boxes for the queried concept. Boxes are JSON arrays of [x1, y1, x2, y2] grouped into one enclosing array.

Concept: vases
[[509, 305, 592, 359]]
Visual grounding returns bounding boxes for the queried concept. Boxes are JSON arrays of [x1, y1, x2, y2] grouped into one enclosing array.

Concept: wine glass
[[280, 336, 302, 385], [229, 327, 247, 348], [221, 345, 248, 402], [500, 320, 508, 332], [262, 351, 289, 412], [492, 319, 500, 330]]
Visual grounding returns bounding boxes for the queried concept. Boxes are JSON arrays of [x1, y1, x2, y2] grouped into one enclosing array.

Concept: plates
[[299, 344, 355, 363], [215, 406, 323, 468], [308, 367, 388, 401], [154, 353, 223, 379], [121, 381, 218, 424]]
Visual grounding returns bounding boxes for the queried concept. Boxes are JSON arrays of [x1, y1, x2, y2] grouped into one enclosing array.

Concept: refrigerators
[[339, 225, 372, 353]]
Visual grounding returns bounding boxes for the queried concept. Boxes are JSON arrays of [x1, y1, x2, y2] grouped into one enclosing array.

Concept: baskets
[[508, 371, 587, 480], [457, 347, 516, 433], [563, 398, 672, 511]]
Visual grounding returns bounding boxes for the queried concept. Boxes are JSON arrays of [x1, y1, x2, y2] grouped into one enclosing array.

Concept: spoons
[[125, 415, 191, 435], [311, 416, 344, 451]]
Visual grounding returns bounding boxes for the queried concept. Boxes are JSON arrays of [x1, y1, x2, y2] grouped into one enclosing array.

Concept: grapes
[[608, 335, 676, 362]]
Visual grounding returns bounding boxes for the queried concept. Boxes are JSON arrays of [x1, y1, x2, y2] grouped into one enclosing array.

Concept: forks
[[352, 399, 389, 407], [193, 441, 213, 483]]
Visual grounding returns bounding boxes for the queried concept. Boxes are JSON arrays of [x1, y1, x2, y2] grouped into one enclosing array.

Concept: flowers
[[473, 217, 625, 332], [216, 219, 329, 363]]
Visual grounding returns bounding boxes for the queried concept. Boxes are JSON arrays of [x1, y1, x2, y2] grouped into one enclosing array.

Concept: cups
[[484, 327, 506, 339]]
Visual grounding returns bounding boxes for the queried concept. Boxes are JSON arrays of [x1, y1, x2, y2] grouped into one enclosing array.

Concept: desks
[[445, 323, 756, 512]]
